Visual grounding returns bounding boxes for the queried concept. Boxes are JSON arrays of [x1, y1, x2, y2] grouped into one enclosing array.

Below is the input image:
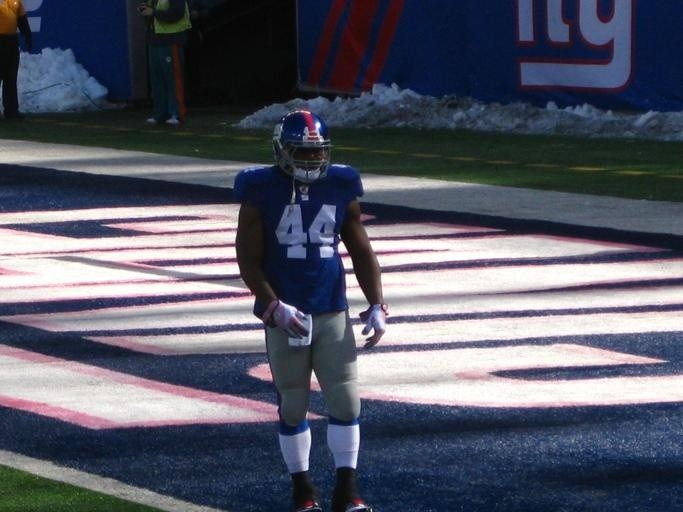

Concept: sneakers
[[331, 498, 373, 512], [292, 491, 323, 512]]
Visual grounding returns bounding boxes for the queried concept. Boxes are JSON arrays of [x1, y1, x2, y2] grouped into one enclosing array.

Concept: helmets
[[272, 110, 329, 183]]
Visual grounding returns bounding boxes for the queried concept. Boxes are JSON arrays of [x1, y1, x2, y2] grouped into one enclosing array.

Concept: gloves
[[262, 299, 310, 339], [362, 304, 386, 349]]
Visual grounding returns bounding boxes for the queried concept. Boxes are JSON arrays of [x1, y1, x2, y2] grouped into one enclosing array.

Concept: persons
[[0, 0, 33, 120], [233, 109, 390, 512], [138, 0, 193, 129]]
